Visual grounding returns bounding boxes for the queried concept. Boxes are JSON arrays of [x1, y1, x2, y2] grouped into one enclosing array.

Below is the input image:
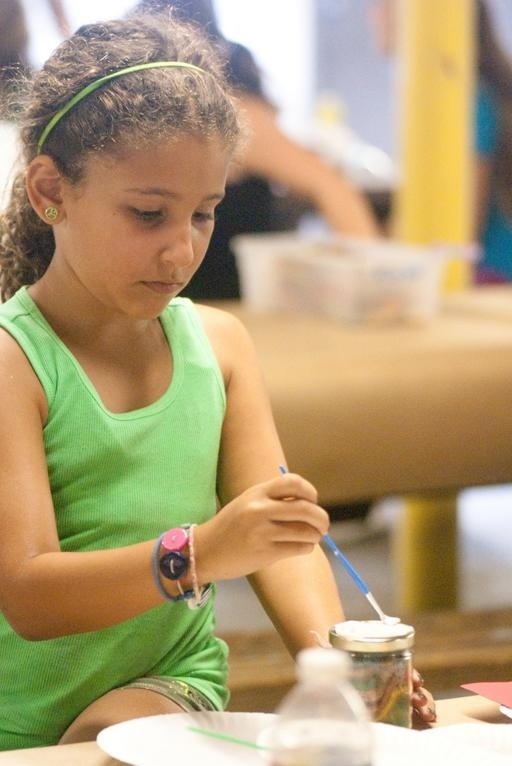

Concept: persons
[[1, 11, 436, 751]]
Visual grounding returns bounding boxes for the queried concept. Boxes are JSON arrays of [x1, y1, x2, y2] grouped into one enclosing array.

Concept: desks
[[205, 288, 512, 509]]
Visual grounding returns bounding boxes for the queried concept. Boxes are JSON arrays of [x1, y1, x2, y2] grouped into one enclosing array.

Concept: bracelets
[[152, 522, 213, 609]]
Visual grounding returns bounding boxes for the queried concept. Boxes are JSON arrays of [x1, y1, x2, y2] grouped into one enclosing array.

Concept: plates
[[95, 712, 422, 766]]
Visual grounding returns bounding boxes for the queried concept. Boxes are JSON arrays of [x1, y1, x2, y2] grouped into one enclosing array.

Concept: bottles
[[329, 620, 415, 728], [265, 645, 373, 764]]
